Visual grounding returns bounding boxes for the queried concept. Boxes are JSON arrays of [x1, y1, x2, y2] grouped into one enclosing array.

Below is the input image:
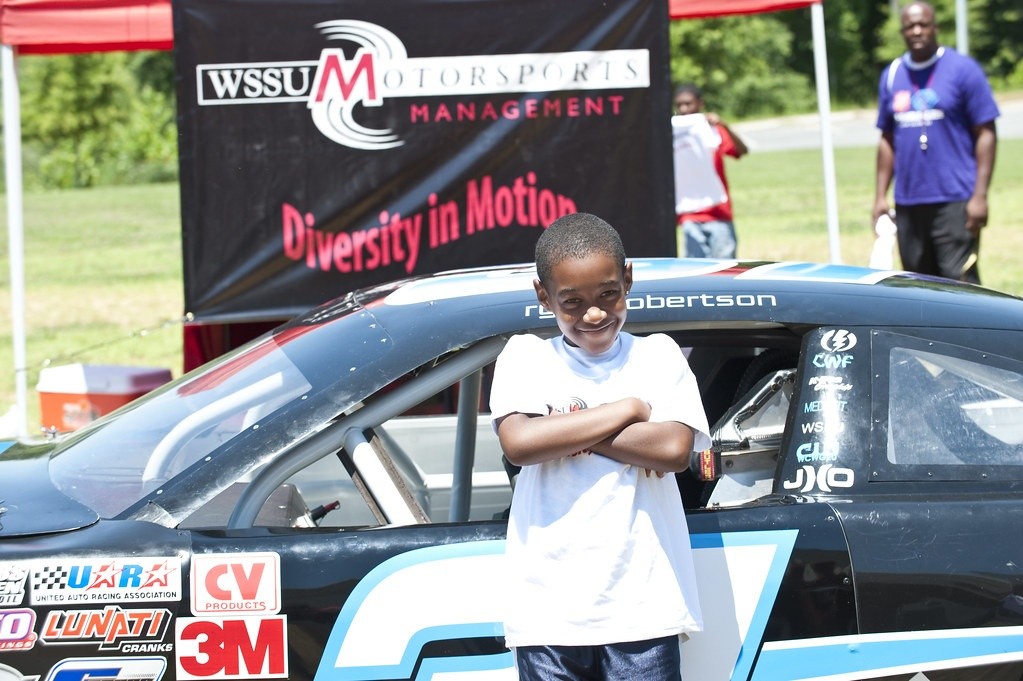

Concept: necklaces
[[910, 65, 936, 152]]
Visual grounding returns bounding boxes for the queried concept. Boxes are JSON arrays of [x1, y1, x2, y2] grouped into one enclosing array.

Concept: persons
[[670, 82, 749, 258], [872, 0, 1002, 285], [489, 211, 715, 681]]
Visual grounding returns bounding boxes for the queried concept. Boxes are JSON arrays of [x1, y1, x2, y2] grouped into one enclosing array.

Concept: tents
[[0, 0, 845, 438]]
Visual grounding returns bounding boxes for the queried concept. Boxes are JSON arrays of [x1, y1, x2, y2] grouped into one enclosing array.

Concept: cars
[[0, 256, 1022, 681]]
